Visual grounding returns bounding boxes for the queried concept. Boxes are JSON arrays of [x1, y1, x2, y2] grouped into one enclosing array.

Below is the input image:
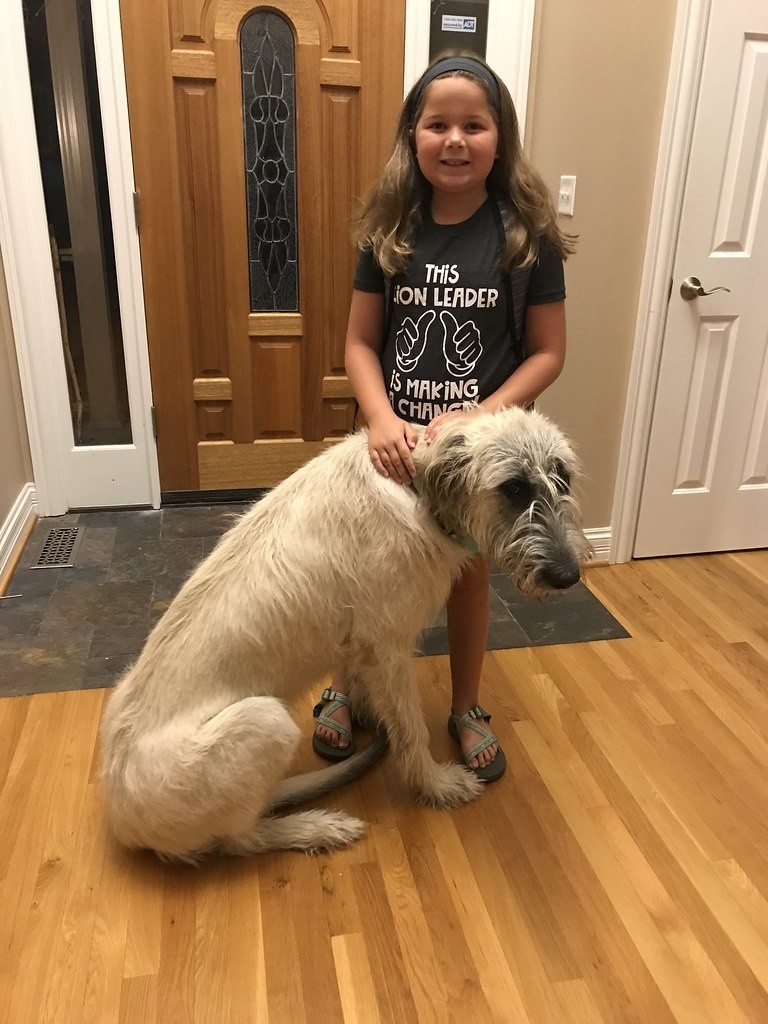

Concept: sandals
[[312, 686, 355, 759], [448, 706, 506, 781]]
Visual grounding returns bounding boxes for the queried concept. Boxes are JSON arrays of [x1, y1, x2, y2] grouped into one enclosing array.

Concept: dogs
[[97, 405, 596, 866]]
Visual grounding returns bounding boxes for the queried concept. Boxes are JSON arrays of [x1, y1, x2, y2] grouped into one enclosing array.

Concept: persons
[[311, 45, 577, 779]]
